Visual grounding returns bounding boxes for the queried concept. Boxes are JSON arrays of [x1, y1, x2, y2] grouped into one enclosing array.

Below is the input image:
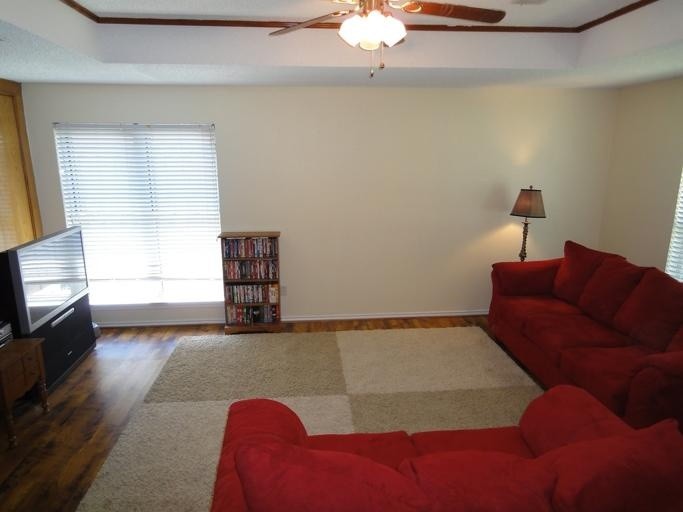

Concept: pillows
[[578, 253, 655, 328], [400, 451, 557, 512], [551, 240, 626, 304], [611, 268, 683, 352], [533, 419, 683, 512], [235, 441, 431, 512]]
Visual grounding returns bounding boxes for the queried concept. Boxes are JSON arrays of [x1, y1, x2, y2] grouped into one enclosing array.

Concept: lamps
[[338, 9, 407, 79], [509, 185, 547, 262]]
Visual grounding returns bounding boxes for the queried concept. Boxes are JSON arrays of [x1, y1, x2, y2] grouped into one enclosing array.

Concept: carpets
[[76, 326, 546, 512]]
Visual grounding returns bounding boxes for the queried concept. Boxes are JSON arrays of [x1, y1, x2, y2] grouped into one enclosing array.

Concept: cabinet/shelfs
[[218, 232, 281, 335], [21, 294, 95, 406]]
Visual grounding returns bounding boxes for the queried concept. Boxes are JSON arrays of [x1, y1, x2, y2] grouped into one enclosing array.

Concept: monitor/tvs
[[0, 226, 89, 335]]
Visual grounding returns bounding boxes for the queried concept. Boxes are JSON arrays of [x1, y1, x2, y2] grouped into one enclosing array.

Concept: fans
[[268, 0, 506, 47]]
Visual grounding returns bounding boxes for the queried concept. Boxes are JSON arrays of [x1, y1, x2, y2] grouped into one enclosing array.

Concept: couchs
[[209, 385, 683, 512], [486, 257, 683, 432]]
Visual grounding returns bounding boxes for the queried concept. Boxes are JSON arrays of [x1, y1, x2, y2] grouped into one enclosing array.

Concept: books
[[219, 236, 280, 326]]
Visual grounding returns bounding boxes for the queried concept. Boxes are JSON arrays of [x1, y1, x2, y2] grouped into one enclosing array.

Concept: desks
[[0, 338, 51, 448]]
